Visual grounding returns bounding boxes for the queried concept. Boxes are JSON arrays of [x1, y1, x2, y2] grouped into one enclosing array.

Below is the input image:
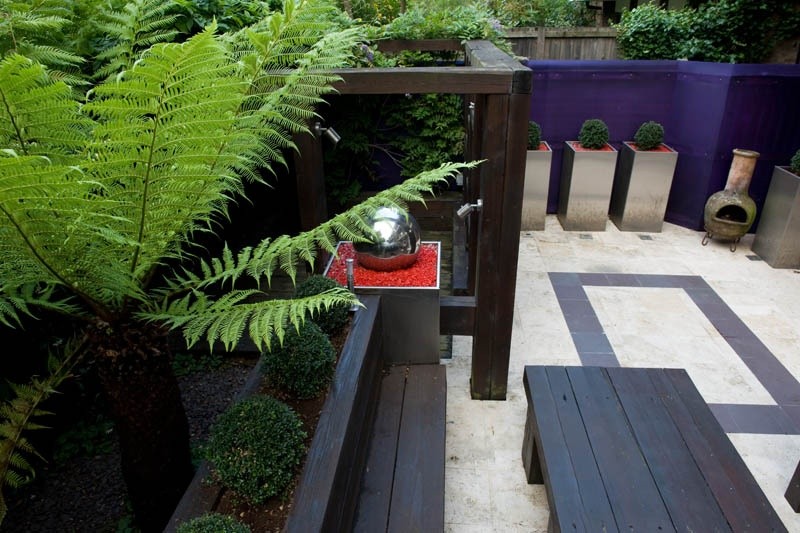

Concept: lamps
[[457, 199, 482, 218], [314, 122, 341, 146]]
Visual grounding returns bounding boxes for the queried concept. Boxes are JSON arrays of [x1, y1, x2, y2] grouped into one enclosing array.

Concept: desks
[[518, 364, 790, 533]]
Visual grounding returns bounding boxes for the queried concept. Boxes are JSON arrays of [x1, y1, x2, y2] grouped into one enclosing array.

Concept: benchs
[[278, 294, 446, 532]]
